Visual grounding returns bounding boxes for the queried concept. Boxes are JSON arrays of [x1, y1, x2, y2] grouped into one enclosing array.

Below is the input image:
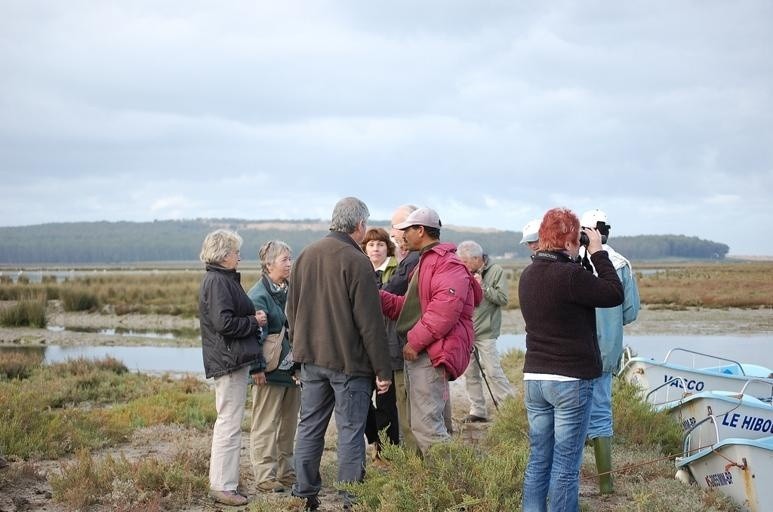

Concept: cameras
[[580, 231, 607, 248]]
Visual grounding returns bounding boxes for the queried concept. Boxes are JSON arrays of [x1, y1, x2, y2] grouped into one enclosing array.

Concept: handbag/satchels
[[261, 325, 286, 374]]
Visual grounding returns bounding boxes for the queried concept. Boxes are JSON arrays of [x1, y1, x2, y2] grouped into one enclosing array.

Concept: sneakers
[[279, 474, 297, 486], [255, 478, 284, 493], [460, 414, 487, 423], [207, 488, 248, 506], [289, 492, 321, 512], [338, 490, 361, 511]]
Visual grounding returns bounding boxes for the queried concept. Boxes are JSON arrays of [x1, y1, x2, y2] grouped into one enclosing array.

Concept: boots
[[366, 441, 395, 471], [593, 435, 616, 494]]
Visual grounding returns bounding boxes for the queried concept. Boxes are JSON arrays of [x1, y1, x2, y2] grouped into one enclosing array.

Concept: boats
[[609, 342, 773, 512]]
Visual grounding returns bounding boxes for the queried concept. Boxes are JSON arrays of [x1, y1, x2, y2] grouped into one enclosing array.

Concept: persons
[[196, 227, 269, 507], [288, 196, 395, 512], [516, 221, 540, 262], [246, 239, 303, 495], [576, 209, 640, 495], [518, 207, 626, 512], [358, 205, 519, 463]]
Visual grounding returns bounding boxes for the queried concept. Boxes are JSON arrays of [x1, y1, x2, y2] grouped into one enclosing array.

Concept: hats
[[519, 220, 541, 245], [392, 207, 442, 230], [578, 208, 610, 230]]
